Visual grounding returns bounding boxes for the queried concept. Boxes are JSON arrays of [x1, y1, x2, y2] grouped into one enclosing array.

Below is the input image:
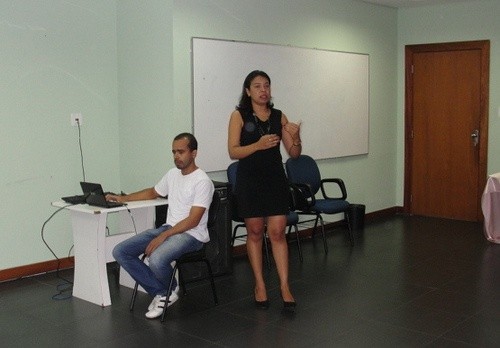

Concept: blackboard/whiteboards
[[190, 36, 369, 173]]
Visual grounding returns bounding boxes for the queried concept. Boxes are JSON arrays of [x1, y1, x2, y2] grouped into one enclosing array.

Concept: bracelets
[[292, 140, 301, 147]]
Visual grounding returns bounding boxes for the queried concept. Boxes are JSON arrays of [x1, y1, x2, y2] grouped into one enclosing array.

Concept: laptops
[[80, 182, 128, 208]]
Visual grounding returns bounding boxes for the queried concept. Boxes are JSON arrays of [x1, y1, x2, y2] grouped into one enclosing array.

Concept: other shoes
[[280, 291, 297, 311], [253, 291, 269, 310]]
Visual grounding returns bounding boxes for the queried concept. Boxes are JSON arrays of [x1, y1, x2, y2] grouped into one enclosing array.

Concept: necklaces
[[252, 110, 272, 137]]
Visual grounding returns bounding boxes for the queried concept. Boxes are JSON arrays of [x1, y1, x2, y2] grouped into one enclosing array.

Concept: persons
[[105, 132, 215, 318], [228, 70, 302, 311]]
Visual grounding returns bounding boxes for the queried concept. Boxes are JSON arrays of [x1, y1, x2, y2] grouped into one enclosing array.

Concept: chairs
[[285, 153, 355, 255], [129, 188, 219, 324], [226, 158, 304, 271]]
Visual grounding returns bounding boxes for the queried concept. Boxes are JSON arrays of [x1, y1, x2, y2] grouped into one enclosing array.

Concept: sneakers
[[145, 291, 179, 318], [148, 284, 178, 310]]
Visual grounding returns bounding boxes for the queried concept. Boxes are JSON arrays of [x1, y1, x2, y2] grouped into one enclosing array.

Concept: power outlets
[[70, 113, 82, 128]]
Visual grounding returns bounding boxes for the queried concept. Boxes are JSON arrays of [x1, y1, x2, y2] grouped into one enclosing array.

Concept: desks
[[51, 194, 181, 307]]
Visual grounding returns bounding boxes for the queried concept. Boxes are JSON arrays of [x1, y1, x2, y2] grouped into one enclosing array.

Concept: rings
[[268, 138, 272, 142]]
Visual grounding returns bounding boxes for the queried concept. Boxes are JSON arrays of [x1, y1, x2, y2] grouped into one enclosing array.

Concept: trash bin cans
[[345, 204, 365, 242]]
